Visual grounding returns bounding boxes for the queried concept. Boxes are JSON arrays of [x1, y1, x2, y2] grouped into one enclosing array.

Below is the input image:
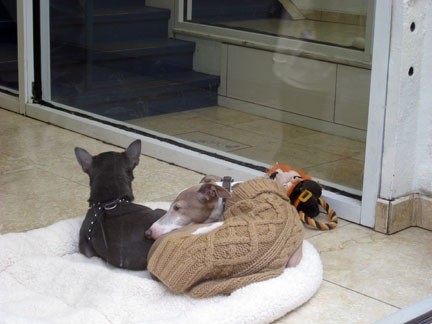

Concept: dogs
[[144, 175, 303, 298], [74, 139, 168, 271]]
